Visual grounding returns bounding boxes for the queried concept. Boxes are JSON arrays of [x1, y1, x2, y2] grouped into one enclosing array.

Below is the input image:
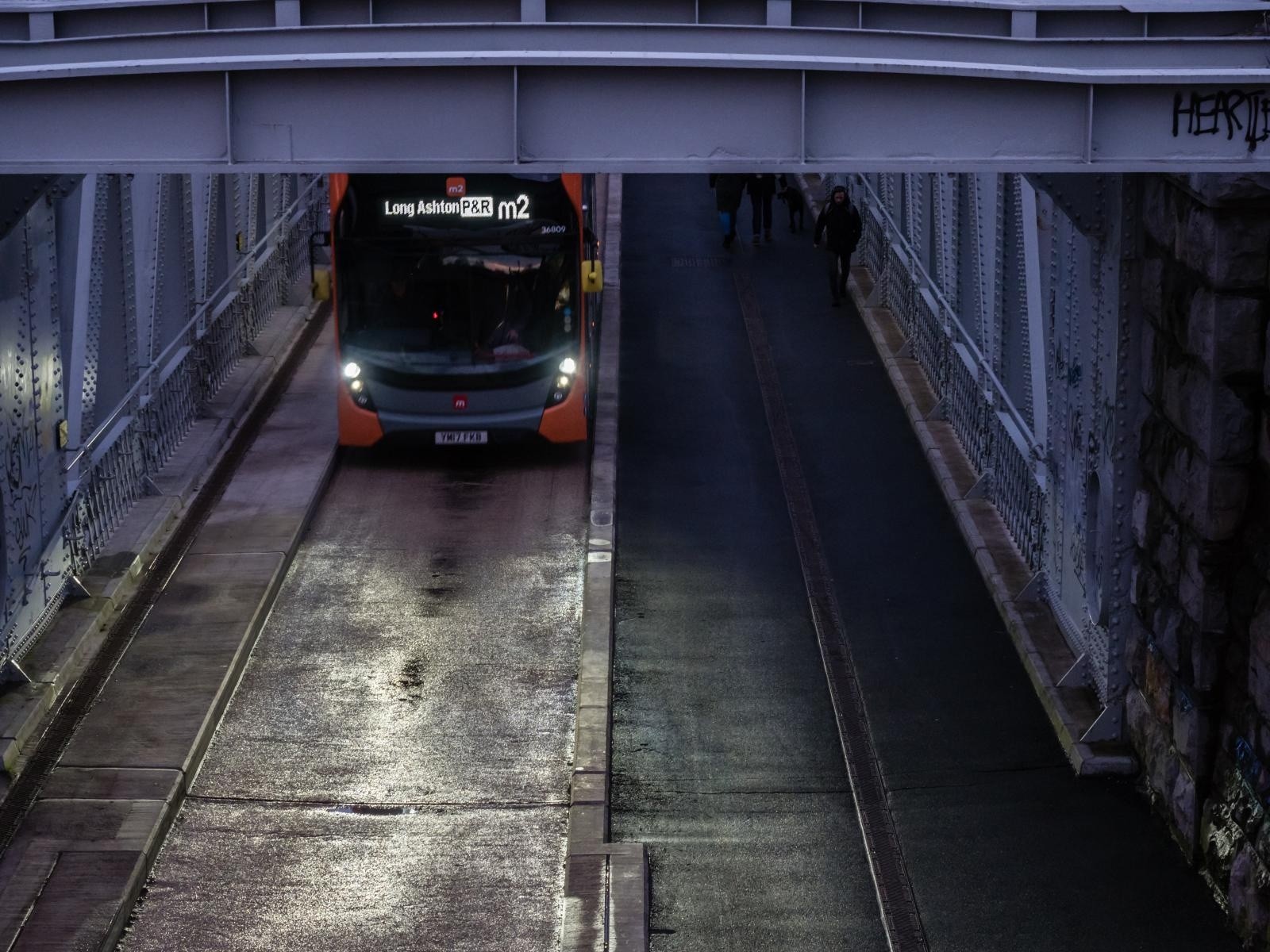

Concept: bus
[[310, 174, 605, 445]]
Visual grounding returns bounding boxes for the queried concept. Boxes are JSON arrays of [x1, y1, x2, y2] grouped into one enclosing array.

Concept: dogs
[[777, 187, 804, 229]]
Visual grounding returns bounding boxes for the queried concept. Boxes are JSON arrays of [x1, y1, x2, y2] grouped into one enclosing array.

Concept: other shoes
[[830, 292, 840, 307], [752, 235, 759, 243], [799, 223, 804, 231], [724, 241, 730, 247], [765, 234, 771, 242], [788, 224, 795, 234], [839, 283, 845, 295]]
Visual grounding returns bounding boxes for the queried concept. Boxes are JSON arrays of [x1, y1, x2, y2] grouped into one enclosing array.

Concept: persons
[[710, 173, 862, 306]]
